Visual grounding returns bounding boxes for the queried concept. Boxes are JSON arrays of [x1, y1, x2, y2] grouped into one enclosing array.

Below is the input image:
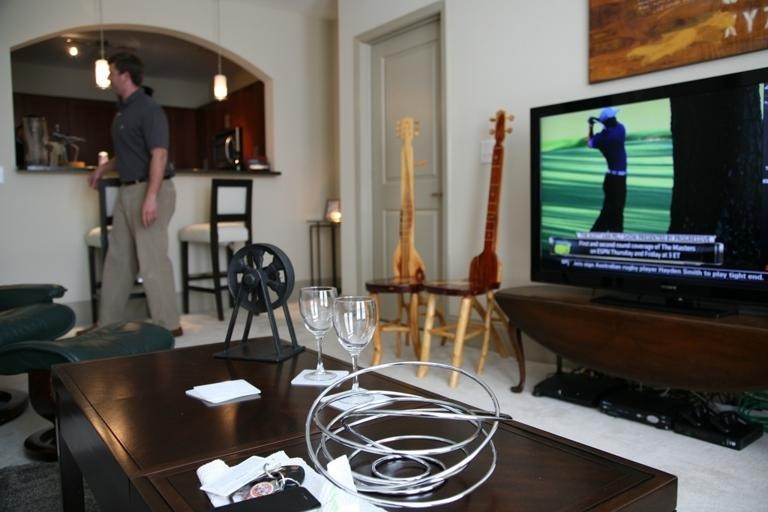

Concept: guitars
[[468, 109, 515, 288], [393, 115, 425, 280]]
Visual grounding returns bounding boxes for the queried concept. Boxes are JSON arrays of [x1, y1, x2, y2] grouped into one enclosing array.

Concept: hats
[[597, 106, 623, 122]]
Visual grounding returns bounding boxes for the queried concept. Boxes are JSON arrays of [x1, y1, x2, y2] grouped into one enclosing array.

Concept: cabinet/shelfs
[[493, 286, 768, 450]]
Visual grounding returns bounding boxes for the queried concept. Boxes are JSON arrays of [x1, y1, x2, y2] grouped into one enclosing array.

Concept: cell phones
[[210, 486, 319, 512]]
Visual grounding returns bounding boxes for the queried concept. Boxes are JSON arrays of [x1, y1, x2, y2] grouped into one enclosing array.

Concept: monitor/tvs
[[529, 67, 767, 320]]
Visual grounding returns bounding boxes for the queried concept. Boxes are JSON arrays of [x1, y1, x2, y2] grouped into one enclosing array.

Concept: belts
[[115, 169, 178, 188], [604, 167, 628, 179]]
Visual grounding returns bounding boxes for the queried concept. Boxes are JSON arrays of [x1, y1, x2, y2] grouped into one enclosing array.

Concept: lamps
[[213, 1, 228, 101], [94, 1, 112, 90]]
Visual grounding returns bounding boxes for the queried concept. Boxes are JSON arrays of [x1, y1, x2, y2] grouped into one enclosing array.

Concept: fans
[[212, 242, 305, 363]]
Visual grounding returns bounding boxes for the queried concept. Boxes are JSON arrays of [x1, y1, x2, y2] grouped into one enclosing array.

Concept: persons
[[76, 51, 185, 338], [586, 107, 628, 233]]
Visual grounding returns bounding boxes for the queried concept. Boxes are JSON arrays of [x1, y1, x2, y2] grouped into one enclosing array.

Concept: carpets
[[1, 461, 101, 512]]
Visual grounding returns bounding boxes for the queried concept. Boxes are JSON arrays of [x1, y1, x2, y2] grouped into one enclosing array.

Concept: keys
[[229, 463, 305, 505]]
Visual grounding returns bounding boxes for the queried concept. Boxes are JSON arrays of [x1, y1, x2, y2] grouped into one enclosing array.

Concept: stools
[[416, 109, 515, 388], [0, 320, 176, 461], [0, 285, 75, 424], [364, 118, 446, 367]]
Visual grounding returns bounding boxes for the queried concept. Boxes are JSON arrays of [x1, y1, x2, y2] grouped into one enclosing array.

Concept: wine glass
[[297, 285, 337, 381], [333, 295, 377, 403]]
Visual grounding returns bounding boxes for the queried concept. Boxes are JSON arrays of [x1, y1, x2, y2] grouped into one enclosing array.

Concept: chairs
[[179, 178, 259, 319], [87, 177, 146, 325]]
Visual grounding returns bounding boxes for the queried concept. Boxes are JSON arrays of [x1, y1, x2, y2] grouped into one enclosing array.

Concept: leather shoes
[[73, 324, 100, 337], [162, 325, 186, 340]]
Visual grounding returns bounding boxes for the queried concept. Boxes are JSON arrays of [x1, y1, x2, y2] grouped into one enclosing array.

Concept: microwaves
[[211, 126, 242, 170]]
